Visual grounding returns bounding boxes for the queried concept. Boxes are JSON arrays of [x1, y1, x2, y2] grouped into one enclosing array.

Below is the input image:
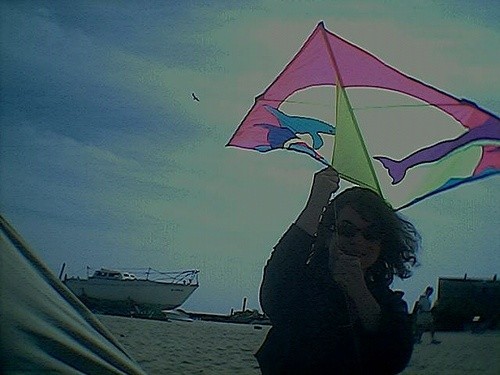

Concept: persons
[[252, 164, 423, 374], [417, 285, 440, 344]]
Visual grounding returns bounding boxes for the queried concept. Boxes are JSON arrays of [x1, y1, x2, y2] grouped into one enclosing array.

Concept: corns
[[218, 21, 499, 209]]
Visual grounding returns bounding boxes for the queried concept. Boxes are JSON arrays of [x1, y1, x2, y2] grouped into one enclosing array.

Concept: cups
[[338, 225, 383, 241]]
